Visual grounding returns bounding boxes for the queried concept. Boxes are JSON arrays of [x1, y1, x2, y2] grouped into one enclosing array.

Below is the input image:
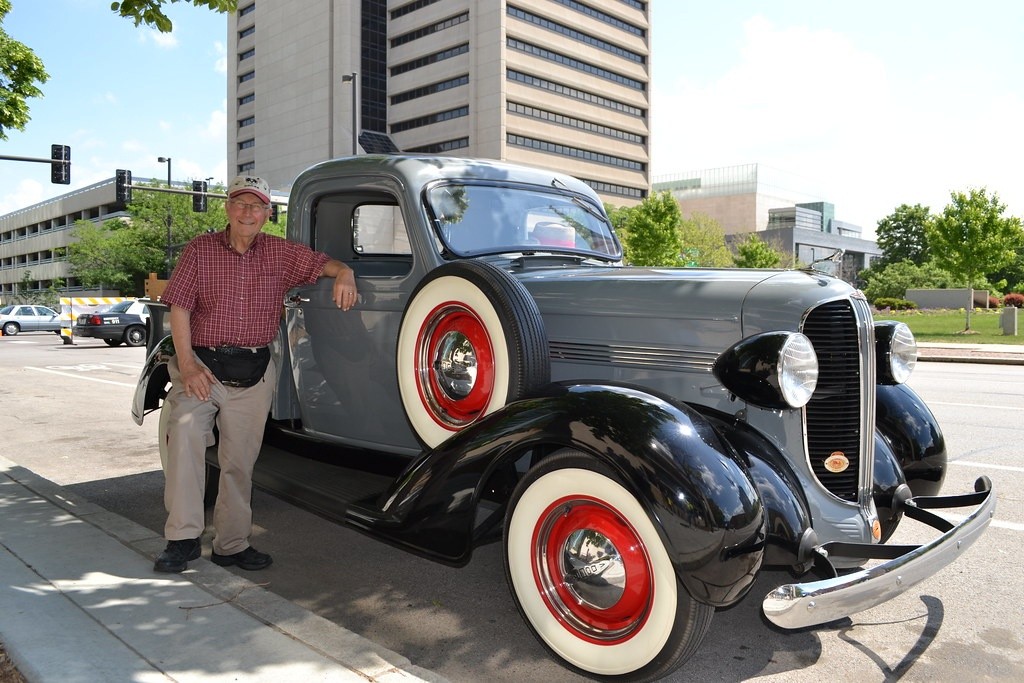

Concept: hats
[[225, 175, 272, 205]]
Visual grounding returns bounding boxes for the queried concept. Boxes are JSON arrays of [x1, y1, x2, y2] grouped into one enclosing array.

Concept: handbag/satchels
[[191, 346, 271, 388]]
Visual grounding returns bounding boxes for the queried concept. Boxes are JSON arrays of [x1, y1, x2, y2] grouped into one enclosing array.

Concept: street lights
[[342, 71, 357, 156], [157, 156, 173, 280]]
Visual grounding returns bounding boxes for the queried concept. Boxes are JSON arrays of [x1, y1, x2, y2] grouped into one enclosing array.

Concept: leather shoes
[[211, 545, 273, 571], [153, 537, 201, 573]]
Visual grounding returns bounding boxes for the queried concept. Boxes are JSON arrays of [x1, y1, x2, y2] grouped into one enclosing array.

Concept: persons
[[152, 175, 359, 572]]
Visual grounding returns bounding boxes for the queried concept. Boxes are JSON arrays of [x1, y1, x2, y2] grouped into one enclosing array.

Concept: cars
[[75, 298, 150, 348], [0, 304, 62, 337], [129, 154, 998, 683]]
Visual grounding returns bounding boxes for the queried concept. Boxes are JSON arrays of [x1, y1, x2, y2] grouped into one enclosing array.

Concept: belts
[[209, 346, 253, 355]]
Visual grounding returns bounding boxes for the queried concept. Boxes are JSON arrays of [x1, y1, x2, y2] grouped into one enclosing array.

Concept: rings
[[348, 293, 353, 295]]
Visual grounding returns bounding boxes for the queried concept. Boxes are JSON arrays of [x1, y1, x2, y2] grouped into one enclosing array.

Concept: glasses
[[229, 200, 270, 211]]
